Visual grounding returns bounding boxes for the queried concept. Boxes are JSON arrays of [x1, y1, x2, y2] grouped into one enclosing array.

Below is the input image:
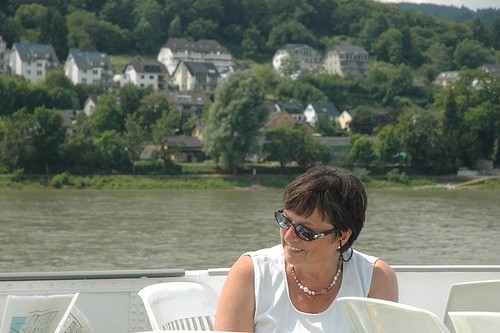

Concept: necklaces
[[290, 252, 342, 295]]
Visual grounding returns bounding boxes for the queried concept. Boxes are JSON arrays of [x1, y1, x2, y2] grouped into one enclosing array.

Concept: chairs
[[138, 280, 216, 331], [334, 280, 500, 332]]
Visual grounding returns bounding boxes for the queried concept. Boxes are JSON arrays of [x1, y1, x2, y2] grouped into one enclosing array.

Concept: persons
[[214, 164, 399, 333]]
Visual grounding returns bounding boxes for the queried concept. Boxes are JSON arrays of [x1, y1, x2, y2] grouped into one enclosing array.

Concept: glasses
[[274, 209, 339, 241]]
